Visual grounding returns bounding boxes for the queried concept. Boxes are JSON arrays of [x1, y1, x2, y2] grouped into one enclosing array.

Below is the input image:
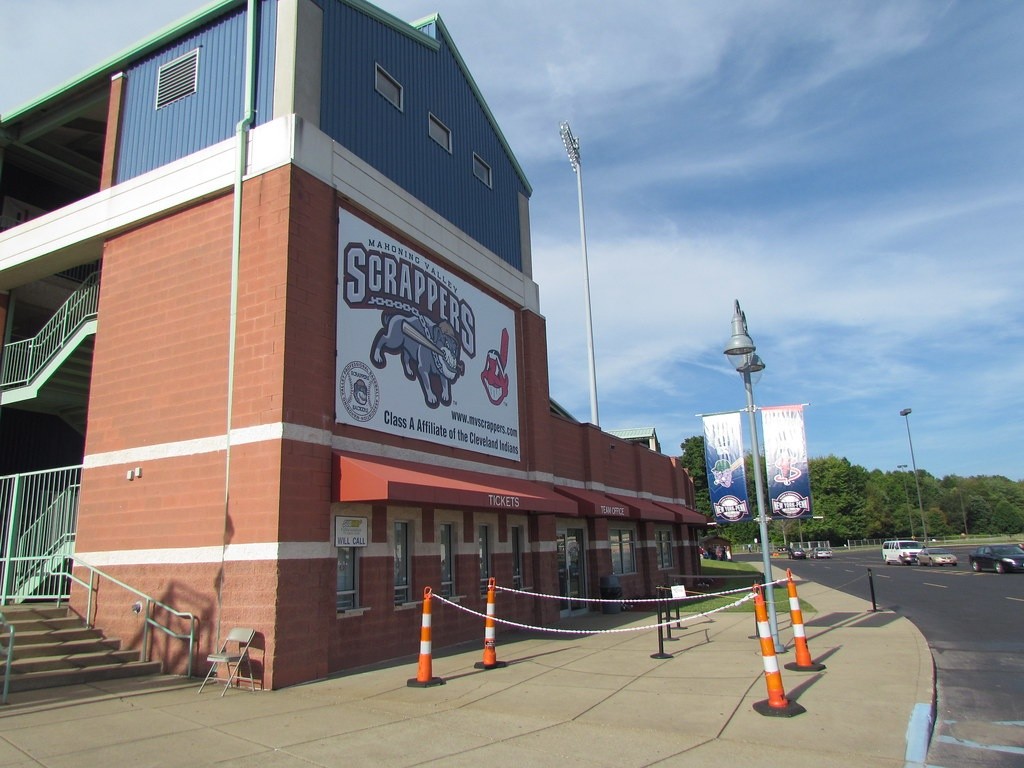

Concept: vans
[[882, 539, 925, 565]]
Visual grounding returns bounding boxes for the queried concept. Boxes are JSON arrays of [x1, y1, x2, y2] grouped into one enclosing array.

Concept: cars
[[789, 548, 807, 559], [916, 547, 958, 566], [968, 544, 1024, 574], [808, 546, 834, 559]]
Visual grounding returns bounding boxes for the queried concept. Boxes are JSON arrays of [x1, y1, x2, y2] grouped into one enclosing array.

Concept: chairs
[[198, 627, 255, 697]]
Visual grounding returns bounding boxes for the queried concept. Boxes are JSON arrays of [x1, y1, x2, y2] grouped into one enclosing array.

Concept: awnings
[[338, 449, 578, 518], [686, 506, 717, 525], [653, 500, 706, 523], [602, 490, 675, 520], [555, 485, 629, 518]]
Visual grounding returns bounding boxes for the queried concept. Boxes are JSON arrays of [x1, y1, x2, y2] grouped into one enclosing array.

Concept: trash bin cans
[[600, 575, 622, 614]]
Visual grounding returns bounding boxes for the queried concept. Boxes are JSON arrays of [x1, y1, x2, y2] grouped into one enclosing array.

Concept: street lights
[[900, 408, 930, 546], [559, 120, 604, 425], [897, 464, 919, 543], [722, 297, 788, 653]]
